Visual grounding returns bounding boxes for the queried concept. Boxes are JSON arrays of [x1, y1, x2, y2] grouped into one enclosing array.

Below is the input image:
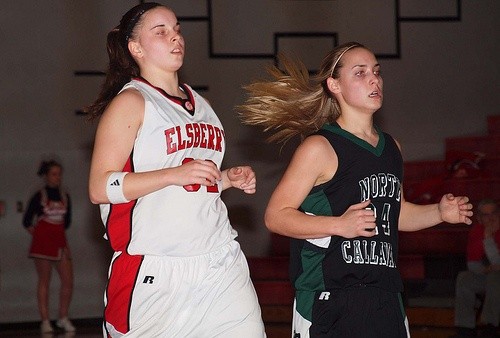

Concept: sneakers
[[41, 320, 54, 332], [57, 317, 75, 331]]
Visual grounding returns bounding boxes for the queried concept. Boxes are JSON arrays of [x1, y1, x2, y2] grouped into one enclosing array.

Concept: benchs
[[244, 113, 500, 326]]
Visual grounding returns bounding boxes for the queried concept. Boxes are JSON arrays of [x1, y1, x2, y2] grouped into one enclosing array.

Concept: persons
[[87, 1, 265, 338], [448, 198, 500, 338], [23, 160, 73, 333], [235, 43, 473, 338]]
